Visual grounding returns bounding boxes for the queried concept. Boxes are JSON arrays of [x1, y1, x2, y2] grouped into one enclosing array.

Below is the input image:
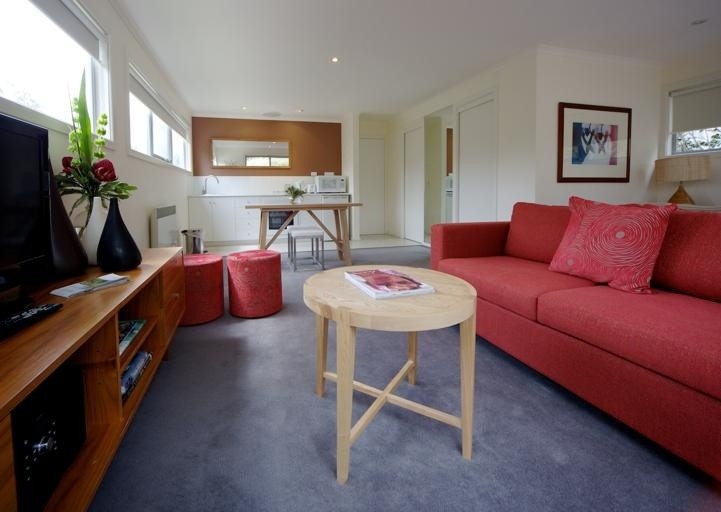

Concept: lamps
[[655, 155, 712, 205]]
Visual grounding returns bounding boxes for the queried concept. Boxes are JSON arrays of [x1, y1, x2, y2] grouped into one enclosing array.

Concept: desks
[[245, 203, 363, 266]]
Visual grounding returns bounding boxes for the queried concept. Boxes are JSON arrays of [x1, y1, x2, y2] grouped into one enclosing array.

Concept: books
[[118, 318, 148, 359], [121, 350, 154, 402], [50, 271, 131, 300], [343, 267, 436, 301]]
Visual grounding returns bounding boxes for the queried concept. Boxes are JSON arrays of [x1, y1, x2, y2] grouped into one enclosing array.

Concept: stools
[[289, 229, 324, 273], [178, 253, 224, 326], [225, 250, 282, 319], [286, 224, 319, 264]]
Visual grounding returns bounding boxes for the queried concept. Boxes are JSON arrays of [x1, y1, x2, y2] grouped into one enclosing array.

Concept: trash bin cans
[[181, 230, 204, 253]]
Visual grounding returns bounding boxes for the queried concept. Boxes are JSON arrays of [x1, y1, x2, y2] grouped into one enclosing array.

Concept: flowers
[[54, 68, 138, 240]]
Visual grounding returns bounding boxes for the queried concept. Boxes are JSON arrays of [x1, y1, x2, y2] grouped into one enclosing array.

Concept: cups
[[295, 182, 315, 194]]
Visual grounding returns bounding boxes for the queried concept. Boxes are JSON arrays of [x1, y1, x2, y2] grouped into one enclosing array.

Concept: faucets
[[204, 175, 219, 194]]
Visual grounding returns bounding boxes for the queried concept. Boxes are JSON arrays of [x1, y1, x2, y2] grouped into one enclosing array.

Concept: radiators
[[148, 205, 179, 248]]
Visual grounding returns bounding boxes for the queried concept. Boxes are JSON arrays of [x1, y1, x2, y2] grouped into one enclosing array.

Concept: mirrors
[[208, 136, 293, 170]]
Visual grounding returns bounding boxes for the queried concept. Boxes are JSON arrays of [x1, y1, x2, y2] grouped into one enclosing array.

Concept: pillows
[[549, 198, 677, 296]]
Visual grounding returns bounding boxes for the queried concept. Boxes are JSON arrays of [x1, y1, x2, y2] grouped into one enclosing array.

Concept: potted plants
[[284, 186, 306, 204]]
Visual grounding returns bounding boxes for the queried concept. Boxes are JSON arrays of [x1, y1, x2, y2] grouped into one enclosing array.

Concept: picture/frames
[[556, 102, 632, 183]]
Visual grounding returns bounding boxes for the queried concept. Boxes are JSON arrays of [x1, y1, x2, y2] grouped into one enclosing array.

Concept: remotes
[[1, 301, 63, 340]]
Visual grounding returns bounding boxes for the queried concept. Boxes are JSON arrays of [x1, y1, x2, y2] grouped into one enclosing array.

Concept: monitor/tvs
[[0, 112, 63, 321]]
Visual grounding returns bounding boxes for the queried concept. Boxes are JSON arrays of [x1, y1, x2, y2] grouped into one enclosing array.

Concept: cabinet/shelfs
[[234, 196, 276, 240], [187, 197, 234, 242], [277, 195, 322, 240], [0, 247, 186, 511], [322, 195, 349, 241]]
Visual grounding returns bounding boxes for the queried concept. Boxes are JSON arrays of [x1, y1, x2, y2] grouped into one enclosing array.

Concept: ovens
[[268, 210, 298, 237]]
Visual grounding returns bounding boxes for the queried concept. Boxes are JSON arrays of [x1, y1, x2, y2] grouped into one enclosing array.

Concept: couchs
[[431, 202, 721, 482]]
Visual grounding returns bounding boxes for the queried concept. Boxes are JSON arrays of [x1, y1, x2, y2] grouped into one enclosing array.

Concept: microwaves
[[313, 176, 346, 194]]
